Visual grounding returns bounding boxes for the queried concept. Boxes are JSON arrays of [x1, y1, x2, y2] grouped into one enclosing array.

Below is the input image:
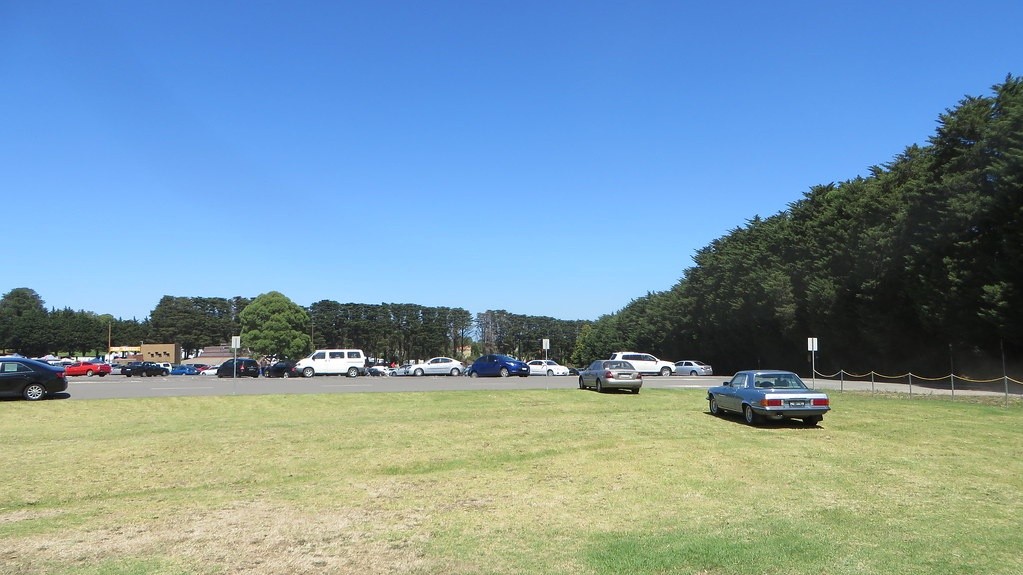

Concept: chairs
[[351, 354, 357, 358], [335, 354, 340, 358], [759, 382, 774, 387]]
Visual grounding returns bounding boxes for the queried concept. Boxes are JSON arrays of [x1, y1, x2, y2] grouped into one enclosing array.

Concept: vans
[[293, 348, 365, 378]]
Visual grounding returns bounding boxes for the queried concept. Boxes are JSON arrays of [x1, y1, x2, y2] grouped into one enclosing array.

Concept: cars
[[65, 361, 112, 377], [673, 361, 713, 376], [120, 361, 170, 377], [0, 356, 68, 401], [404, 356, 464, 377], [263, 360, 298, 379], [31, 358, 73, 371], [468, 354, 530, 378], [579, 360, 643, 394], [526, 360, 570, 376], [463, 366, 471, 376], [705, 369, 831, 427], [148, 361, 220, 376], [365, 366, 397, 377], [109, 363, 124, 375], [569, 368, 586, 377]]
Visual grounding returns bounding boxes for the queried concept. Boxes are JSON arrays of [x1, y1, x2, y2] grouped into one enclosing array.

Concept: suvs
[[609, 351, 676, 377], [216, 358, 260, 378]]
